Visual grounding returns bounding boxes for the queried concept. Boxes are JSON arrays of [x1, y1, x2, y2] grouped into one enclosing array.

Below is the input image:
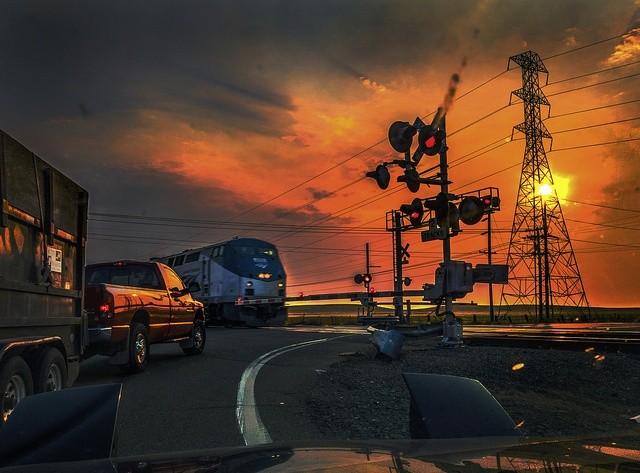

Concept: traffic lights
[[362, 273, 371, 282], [424, 192, 449, 221], [401, 198, 423, 225], [419, 127, 443, 155], [481, 195, 493, 208]]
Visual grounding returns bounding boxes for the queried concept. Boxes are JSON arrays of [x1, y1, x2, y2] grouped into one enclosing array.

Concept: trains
[[149, 235, 288, 327]]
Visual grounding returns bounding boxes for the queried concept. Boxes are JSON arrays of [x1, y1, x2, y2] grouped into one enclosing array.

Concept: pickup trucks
[[85, 259, 206, 373]]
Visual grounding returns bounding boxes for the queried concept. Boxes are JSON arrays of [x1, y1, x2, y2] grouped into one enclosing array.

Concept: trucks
[[0, 128, 89, 424]]
